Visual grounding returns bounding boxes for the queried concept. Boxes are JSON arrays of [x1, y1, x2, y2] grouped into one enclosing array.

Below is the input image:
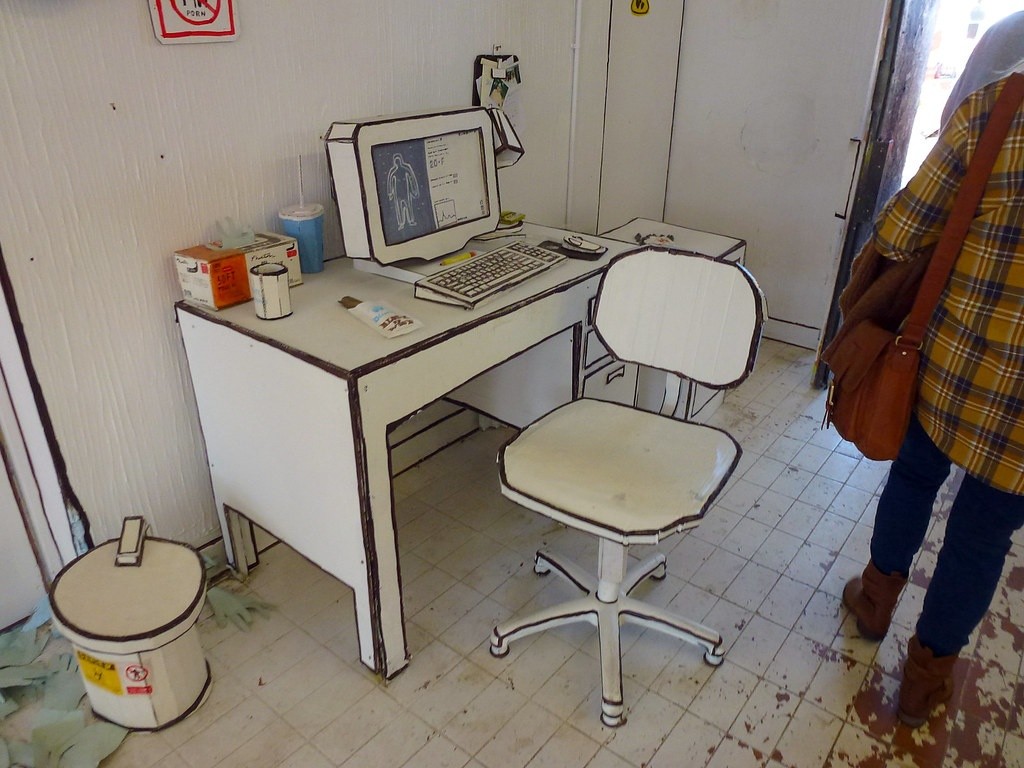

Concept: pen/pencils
[[439, 252, 476, 265]]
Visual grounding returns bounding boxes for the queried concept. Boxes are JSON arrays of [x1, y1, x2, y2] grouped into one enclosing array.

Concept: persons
[[842, 64, 1024, 729], [940, 11, 1023, 131]]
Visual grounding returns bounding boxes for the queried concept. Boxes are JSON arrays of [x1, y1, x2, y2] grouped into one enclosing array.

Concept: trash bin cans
[[49, 515, 212, 729]]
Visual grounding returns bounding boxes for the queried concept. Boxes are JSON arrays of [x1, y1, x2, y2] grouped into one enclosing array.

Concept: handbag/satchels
[[821, 312, 921, 461]]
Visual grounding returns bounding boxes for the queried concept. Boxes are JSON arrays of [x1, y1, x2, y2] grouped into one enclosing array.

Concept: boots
[[842, 558, 910, 642], [899, 632, 953, 723]]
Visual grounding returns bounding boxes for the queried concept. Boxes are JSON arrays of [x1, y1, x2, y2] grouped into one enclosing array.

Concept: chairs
[[487, 248, 769, 728]]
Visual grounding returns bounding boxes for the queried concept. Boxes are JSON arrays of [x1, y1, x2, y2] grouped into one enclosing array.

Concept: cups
[[278, 203, 325, 274], [250, 262, 292, 320]]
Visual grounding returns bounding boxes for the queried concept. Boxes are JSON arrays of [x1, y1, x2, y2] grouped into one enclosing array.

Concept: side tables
[[600, 213, 747, 424]]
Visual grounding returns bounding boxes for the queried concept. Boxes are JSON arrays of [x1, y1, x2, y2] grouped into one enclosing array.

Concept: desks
[[173, 223, 651, 681]]
